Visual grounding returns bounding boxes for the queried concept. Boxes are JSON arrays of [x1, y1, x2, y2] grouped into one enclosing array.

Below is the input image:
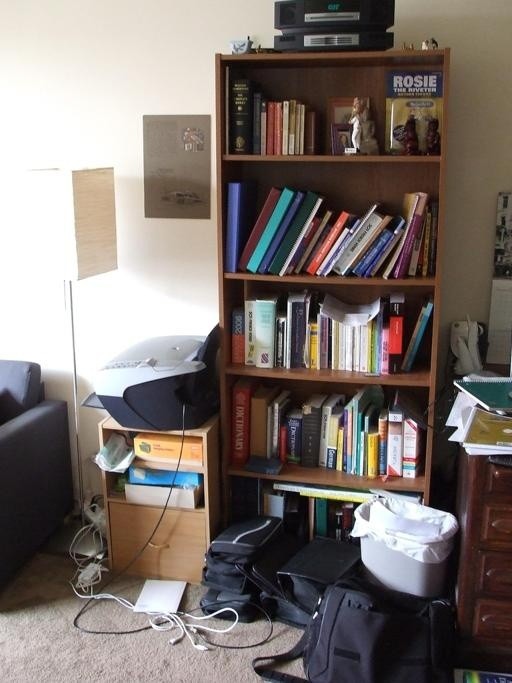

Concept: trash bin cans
[[356, 496, 457, 598]]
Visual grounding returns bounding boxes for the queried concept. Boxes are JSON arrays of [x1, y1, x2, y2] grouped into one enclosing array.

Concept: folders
[[263, 489, 285, 518]]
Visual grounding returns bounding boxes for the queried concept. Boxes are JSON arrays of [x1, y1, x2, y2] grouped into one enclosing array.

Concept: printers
[[81, 323, 219, 431]]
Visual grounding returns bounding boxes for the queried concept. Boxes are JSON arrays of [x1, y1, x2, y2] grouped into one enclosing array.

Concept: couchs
[[0, 358, 75, 587]]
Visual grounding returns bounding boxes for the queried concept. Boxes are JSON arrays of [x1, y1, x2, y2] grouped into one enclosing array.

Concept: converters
[[78, 562, 101, 581]]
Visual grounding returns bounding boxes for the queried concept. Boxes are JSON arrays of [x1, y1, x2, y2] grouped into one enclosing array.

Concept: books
[[222, 64, 315, 156], [231, 381, 421, 481], [231, 294, 432, 375], [446, 374, 512, 456], [224, 182, 438, 278]]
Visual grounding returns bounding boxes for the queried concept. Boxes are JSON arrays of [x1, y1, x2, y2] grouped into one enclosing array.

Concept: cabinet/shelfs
[[213, 47, 449, 542], [98, 413, 218, 587], [453, 442, 512, 656]]
